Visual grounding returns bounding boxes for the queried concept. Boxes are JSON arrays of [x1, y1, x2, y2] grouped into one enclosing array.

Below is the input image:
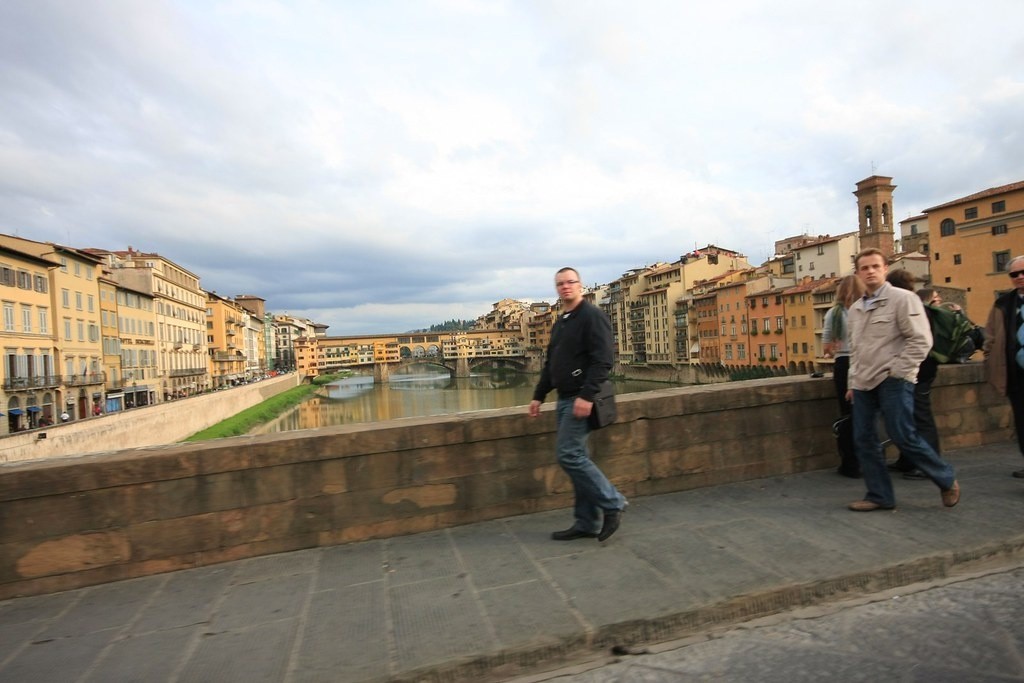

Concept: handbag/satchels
[[588, 380, 617, 428]]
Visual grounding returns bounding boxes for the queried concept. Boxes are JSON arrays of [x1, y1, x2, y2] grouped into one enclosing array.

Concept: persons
[[822, 275, 868, 479], [528, 267, 630, 542], [983, 255, 1024, 478], [94, 405, 100, 416], [886, 268, 975, 480], [18, 412, 70, 432], [167, 368, 287, 404], [846, 248, 961, 511]]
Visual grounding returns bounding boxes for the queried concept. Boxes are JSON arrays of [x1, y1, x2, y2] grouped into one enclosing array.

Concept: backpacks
[[921, 300, 987, 366]]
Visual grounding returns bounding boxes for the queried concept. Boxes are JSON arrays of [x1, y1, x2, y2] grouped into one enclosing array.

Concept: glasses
[[1008, 270, 1024, 277], [556, 280, 579, 286]]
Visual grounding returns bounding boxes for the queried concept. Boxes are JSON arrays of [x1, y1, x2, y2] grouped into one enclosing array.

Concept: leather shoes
[[598, 497, 629, 542], [551, 526, 601, 541]]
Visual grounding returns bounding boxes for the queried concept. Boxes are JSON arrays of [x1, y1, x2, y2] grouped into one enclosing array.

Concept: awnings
[[9, 410, 24, 415], [28, 407, 42, 412]]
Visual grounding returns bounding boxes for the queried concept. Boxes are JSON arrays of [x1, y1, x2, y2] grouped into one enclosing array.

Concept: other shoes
[[903, 468, 927, 480], [1013, 469, 1024, 477], [849, 499, 896, 511], [941, 478, 960, 508], [886, 460, 905, 472]]
